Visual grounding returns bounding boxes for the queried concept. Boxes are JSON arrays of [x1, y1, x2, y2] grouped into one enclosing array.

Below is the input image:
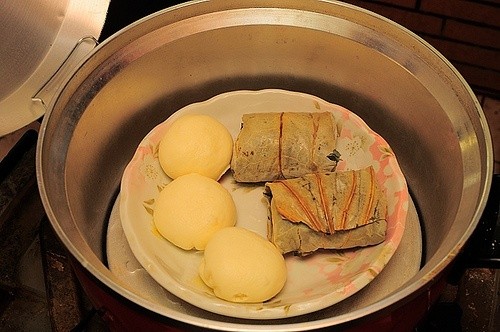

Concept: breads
[[153, 115, 286, 303]]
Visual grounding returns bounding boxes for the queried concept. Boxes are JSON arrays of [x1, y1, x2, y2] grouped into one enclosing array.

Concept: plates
[[120, 89, 408, 319], [0, 1, 110, 137]]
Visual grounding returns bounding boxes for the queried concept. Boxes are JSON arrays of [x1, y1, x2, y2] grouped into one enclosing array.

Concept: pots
[[31, 0, 493, 332]]
